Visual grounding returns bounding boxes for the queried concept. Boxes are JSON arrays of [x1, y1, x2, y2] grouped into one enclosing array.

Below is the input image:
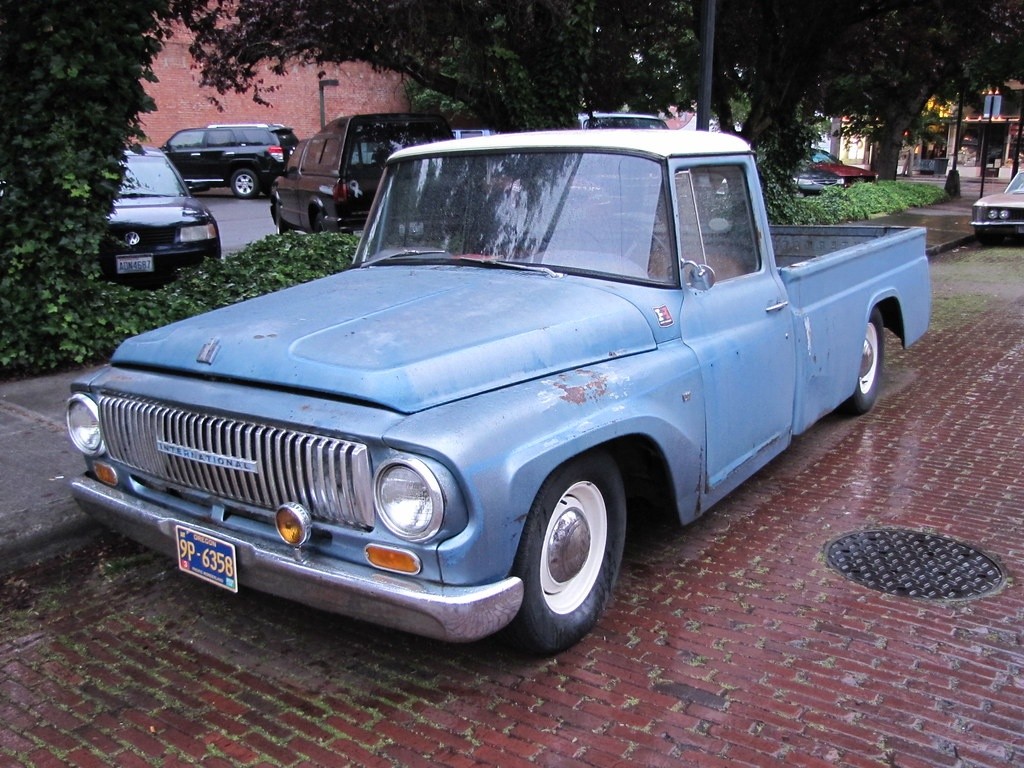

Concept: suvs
[[158, 123, 301, 200]]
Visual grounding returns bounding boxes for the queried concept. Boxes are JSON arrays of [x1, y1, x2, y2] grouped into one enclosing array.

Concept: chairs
[[643, 252, 745, 283]]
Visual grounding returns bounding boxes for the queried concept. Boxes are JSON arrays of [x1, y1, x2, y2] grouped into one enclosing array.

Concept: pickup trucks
[[270, 113, 458, 236], [65, 130, 934, 654]]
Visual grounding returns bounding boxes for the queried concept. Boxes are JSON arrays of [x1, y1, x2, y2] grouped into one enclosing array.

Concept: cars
[[98, 143, 222, 288], [970, 170, 1024, 245], [806, 146, 879, 190], [792, 164, 846, 200], [452, 129, 499, 141], [577, 110, 670, 131]]
